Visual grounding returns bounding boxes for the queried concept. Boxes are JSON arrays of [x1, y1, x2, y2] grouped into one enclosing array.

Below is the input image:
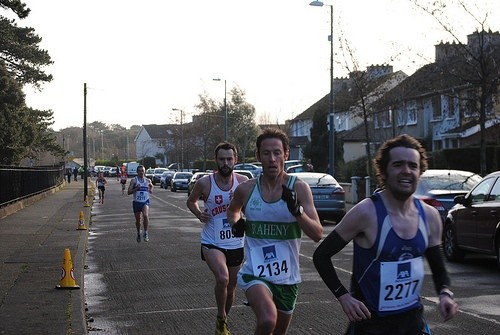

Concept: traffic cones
[[83, 195, 90, 207], [88, 177, 96, 200], [56, 248, 81, 290], [76, 211, 87, 230]]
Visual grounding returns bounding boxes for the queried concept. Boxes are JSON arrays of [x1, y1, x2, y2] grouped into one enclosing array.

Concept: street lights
[[171, 108, 183, 172], [212, 79, 227, 141], [309, 0, 336, 180]]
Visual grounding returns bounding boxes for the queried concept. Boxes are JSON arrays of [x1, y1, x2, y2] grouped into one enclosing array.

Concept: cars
[[188, 171, 215, 200], [373, 168, 491, 216], [232, 160, 264, 180], [159, 171, 175, 190], [171, 171, 194, 192], [284, 160, 314, 173], [152, 167, 170, 185], [145, 169, 155, 180], [287, 172, 346, 224], [77, 165, 122, 177], [442, 170, 500, 267]]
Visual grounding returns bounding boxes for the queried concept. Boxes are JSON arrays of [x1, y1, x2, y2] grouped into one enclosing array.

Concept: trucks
[[127, 162, 139, 178], [169, 162, 199, 174]]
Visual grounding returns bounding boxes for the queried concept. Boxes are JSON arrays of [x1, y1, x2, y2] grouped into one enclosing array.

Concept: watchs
[[292, 204, 304, 215]]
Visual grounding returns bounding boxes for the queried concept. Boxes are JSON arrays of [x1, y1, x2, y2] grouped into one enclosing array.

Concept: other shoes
[[215, 315, 233, 335], [143, 231, 149, 242], [137, 234, 141, 243]]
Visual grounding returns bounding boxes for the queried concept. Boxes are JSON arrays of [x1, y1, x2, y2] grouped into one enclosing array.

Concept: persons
[[186, 142, 249, 335], [96, 172, 107, 204], [227, 127, 323, 335], [128, 165, 154, 243], [312, 133, 458, 335], [66, 165, 84, 183], [120, 170, 127, 196]]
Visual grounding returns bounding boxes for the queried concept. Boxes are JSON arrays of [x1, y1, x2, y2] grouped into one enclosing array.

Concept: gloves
[[231, 217, 246, 239], [280, 183, 303, 216]]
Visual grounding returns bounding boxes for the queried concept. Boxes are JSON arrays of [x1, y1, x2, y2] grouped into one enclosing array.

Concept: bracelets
[[440, 288, 455, 299]]
